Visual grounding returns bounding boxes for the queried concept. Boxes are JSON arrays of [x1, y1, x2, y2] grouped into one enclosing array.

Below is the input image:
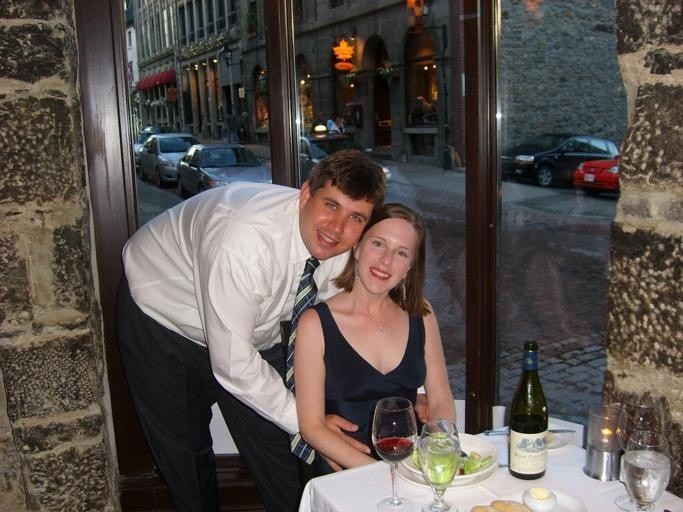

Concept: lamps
[[218, 43, 244, 77]]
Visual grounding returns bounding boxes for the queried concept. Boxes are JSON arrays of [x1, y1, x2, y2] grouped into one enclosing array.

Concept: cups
[[583, 405, 619, 482]]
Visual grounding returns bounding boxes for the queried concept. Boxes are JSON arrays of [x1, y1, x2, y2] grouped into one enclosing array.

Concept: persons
[[328, 116, 346, 134], [113, 147, 388, 512], [291, 202, 458, 478], [223, 110, 240, 143], [309, 112, 326, 133], [144, 122, 170, 134]]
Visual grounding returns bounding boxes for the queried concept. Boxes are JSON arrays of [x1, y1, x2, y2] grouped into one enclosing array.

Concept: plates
[[393, 431, 500, 490], [509, 420, 571, 450], [481, 489, 587, 512]]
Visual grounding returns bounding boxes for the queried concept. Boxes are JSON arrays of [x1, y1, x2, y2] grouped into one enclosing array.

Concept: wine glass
[[416, 420, 461, 512], [370, 396, 419, 510], [611, 392, 673, 511]]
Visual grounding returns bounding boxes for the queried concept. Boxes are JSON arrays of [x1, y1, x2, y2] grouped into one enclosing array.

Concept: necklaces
[[344, 293, 397, 335]]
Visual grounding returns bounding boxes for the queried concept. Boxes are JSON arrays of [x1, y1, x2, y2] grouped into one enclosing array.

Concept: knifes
[[481, 428, 576, 433]]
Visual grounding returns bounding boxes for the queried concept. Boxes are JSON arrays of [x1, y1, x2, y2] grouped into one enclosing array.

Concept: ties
[[287, 255, 325, 465]]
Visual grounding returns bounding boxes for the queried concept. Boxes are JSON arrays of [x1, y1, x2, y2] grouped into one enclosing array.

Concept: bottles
[[505, 337, 549, 478]]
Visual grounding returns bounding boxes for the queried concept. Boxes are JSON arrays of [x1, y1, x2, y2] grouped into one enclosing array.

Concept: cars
[[502, 130, 620, 189], [574, 156, 620, 197], [132, 125, 273, 204], [299, 132, 392, 199]]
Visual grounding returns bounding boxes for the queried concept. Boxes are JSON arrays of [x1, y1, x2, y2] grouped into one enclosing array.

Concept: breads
[[471, 500, 532, 512]]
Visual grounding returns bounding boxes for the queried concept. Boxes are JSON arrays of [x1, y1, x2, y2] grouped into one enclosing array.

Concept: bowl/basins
[[522, 487, 556, 512]]
